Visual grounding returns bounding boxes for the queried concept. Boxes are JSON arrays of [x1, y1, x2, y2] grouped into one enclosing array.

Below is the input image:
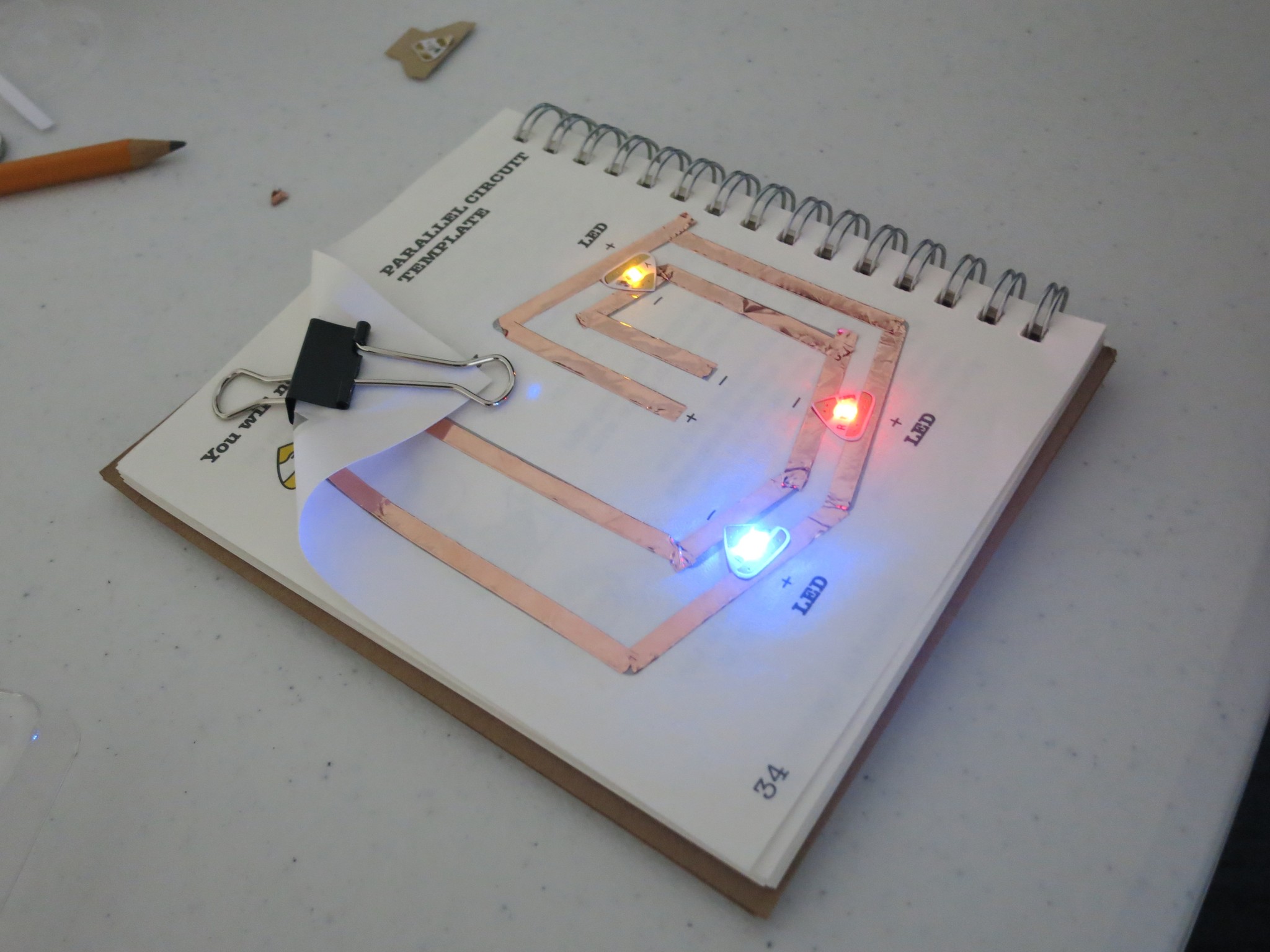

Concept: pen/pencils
[[0, 139, 186, 197]]
